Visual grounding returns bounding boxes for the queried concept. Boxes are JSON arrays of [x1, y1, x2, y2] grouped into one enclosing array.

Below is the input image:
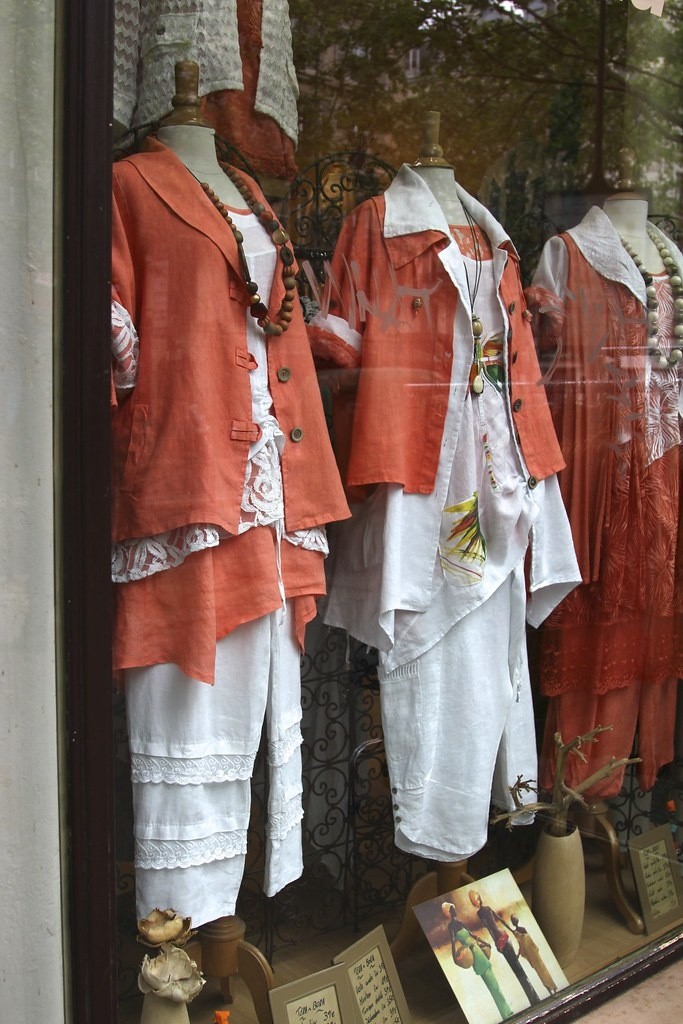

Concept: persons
[[527, 196, 683, 789], [307, 163, 584, 876], [107, 125, 353, 944]]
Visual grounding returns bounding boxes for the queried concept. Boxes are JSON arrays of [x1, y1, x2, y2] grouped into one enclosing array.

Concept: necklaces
[[616, 225, 683, 370], [446, 200, 483, 399], [183, 153, 297, 338]]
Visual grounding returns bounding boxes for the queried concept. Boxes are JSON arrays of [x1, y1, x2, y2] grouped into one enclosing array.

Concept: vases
[[538, 820, 587, 969]]
